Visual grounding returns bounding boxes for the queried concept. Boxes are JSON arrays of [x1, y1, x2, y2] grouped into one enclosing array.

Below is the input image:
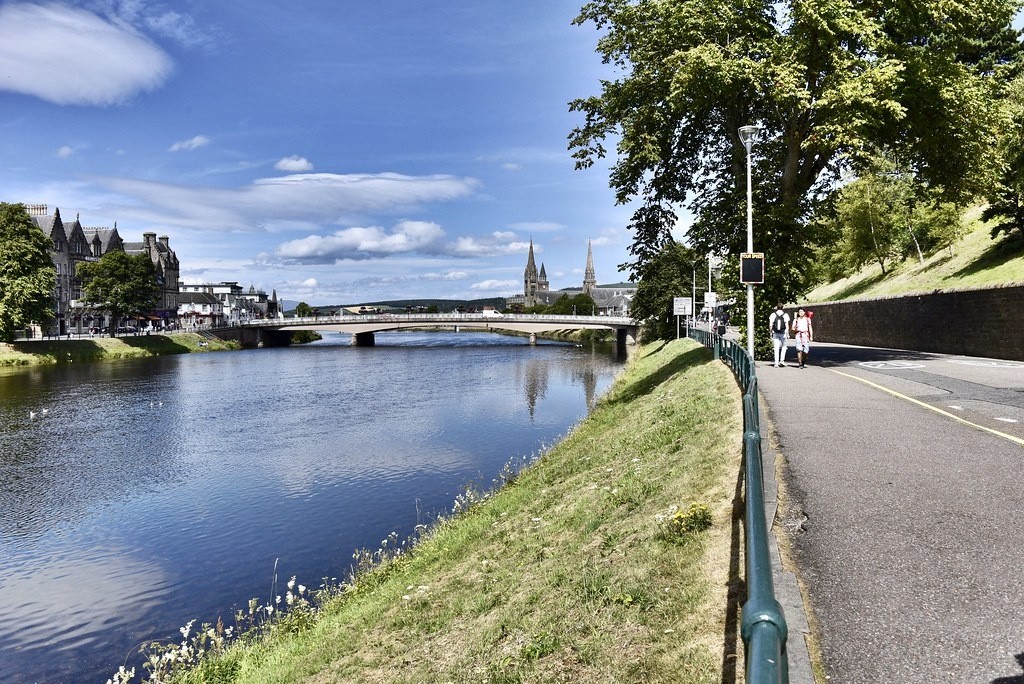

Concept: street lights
[[737, 122, 763, 366]]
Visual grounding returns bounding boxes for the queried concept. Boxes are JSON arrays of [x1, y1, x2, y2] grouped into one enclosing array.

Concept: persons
[[791, 308, 813, 369], [198, 341, 208, 347], [769, 303, 790, 368]]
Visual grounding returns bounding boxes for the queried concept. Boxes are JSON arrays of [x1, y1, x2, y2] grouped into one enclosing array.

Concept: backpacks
[[773, 311, 786, 333]]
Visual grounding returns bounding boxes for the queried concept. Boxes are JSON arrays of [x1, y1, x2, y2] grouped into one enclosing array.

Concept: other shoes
[[779, 360, 786, 367], [774, 363, 779, 368], [799, 361, 804, 369]]
